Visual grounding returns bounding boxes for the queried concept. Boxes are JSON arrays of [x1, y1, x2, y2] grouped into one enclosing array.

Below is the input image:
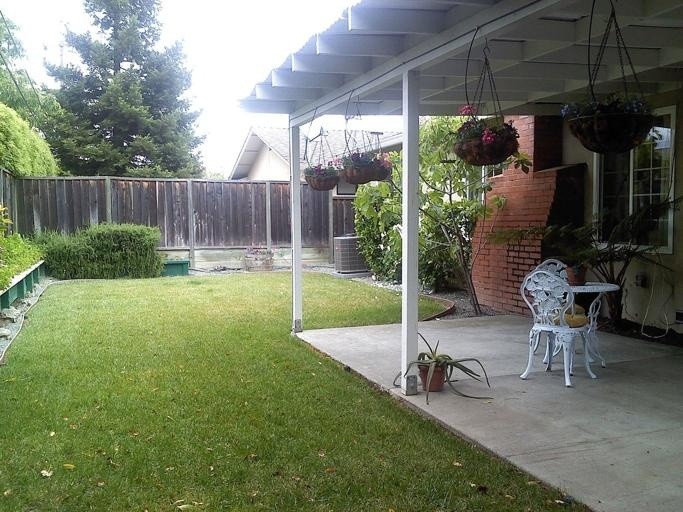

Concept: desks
[[535, 281, 620, 376]]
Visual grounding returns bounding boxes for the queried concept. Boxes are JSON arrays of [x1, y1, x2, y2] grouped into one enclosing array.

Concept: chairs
[[519, 258, 597, 387]]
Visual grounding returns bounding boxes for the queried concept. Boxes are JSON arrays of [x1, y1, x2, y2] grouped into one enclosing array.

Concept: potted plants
[[393, 330, 493, 405]]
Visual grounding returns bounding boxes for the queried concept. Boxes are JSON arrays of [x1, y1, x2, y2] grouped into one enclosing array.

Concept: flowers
[[301, 151, 393, 181], [560, 91, 654, 120], [450, 101, 532, 175]]
